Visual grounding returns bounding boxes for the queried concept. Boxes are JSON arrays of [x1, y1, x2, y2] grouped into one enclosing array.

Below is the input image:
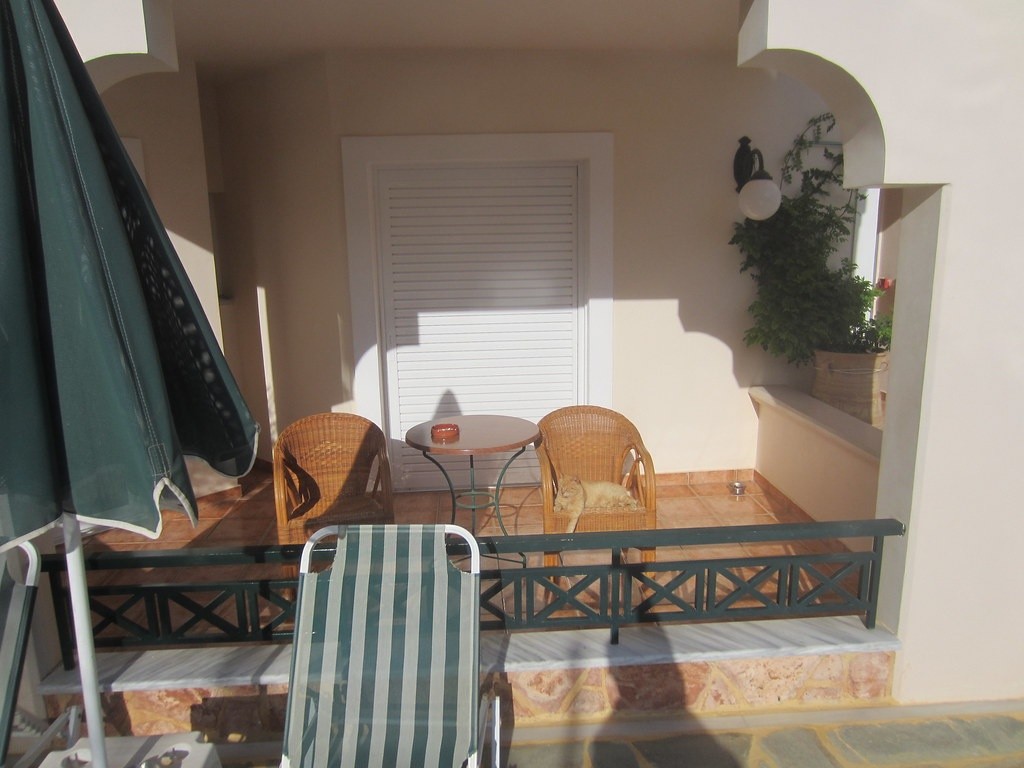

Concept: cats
[[553, 472, 638, 533]]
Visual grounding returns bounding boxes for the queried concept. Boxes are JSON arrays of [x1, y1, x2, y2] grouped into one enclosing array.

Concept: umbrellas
[[0, 0, 261, 768]]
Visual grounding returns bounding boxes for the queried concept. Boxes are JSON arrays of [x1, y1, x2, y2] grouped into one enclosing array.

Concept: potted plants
[[726, 113, 893, 427]]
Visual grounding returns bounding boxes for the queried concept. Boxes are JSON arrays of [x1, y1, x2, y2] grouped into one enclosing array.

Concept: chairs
[[273, 411, 394, 622], [534, 404, 657, 605], [1, 538, 80, 768], [274, 525, 502, 767]]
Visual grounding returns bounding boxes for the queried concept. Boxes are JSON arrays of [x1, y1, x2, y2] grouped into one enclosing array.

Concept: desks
[[407, 413, 541, 569]]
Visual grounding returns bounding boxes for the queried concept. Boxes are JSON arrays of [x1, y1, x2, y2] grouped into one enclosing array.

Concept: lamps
[[733, 136, 780, 222]]
